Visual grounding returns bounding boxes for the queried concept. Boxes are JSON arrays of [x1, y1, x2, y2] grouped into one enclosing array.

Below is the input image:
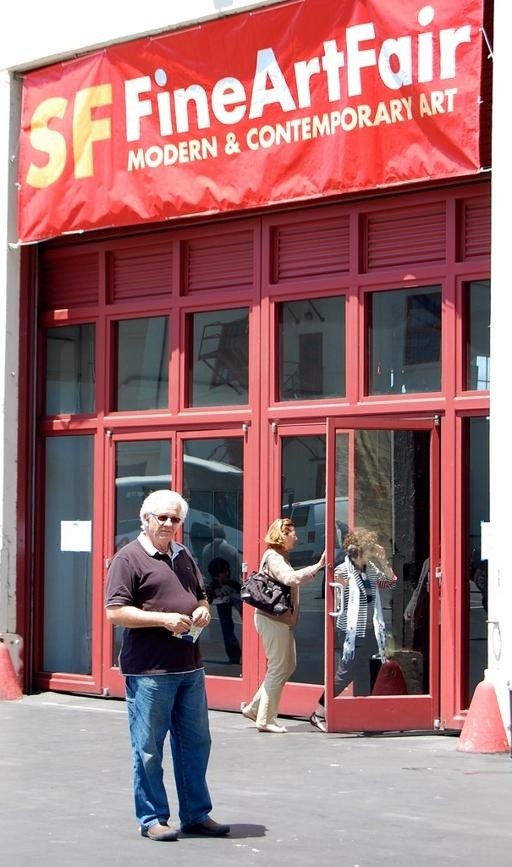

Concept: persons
[[308, 524, 398, 735], [403, 555, 430, 695], [241, 517, 325, 734], [103, 487, 231, 844]]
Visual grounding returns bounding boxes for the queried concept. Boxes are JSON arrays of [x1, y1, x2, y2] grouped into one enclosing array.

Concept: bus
[[115, 456, 241, 599]]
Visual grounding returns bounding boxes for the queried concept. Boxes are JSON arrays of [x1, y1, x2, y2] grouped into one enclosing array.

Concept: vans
[[282, 494, 347, 564]]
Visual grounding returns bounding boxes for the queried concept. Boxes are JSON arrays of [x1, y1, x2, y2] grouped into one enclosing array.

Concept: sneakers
[[242, 704, 288, 733], [310, 712, 326, 733], [141, 822, 178, 841], [181, 817, 231, 835]]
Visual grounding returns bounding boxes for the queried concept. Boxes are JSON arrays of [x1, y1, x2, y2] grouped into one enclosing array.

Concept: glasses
[[150, 512, 181, 523]]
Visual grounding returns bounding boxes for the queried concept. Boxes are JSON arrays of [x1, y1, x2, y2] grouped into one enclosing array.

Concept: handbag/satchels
[[240, 553, 291, 616]]
